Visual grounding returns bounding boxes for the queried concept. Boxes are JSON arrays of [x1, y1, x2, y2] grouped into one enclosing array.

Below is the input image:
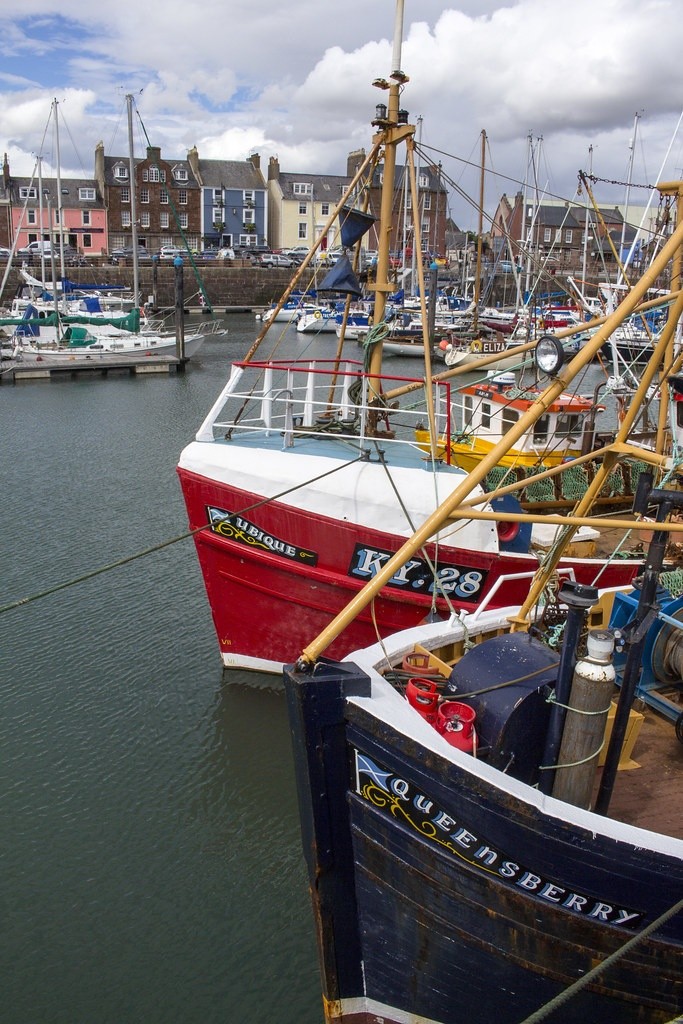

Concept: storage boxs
[[529, 511, 601, 559], [598, 702, 645, 768]]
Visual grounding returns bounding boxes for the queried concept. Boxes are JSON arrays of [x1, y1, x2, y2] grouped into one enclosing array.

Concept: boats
[[282, 224, 683, 1023], [0, 89, 683, 470], [170, 0, 680, 680]]
